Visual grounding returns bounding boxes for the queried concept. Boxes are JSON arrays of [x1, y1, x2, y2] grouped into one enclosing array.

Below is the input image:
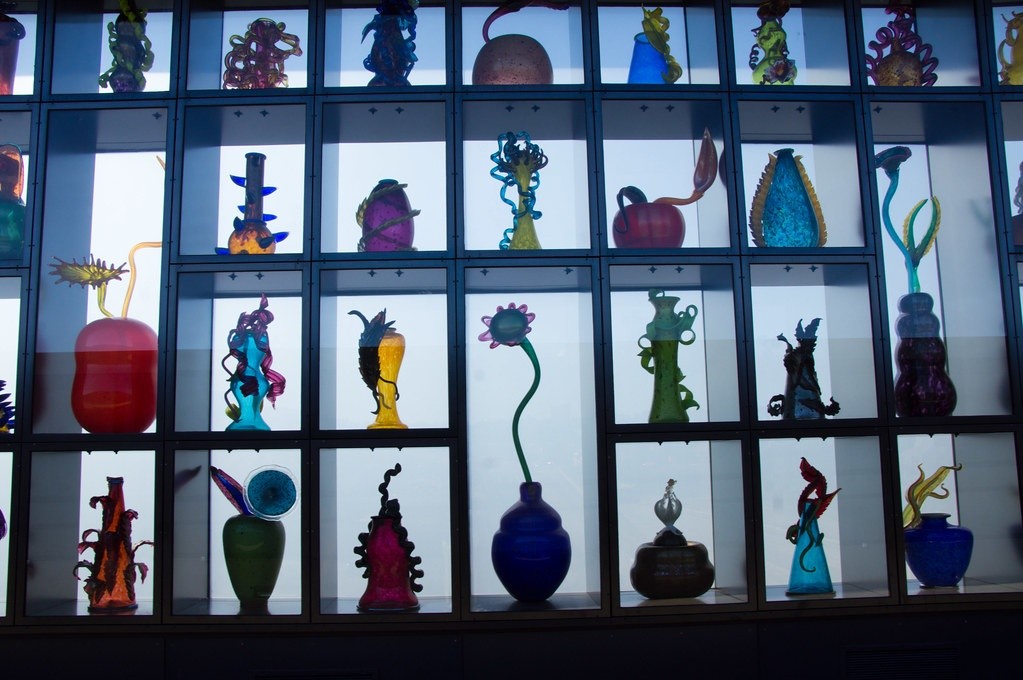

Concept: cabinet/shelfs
[[0, 1, 1022, 636]]
